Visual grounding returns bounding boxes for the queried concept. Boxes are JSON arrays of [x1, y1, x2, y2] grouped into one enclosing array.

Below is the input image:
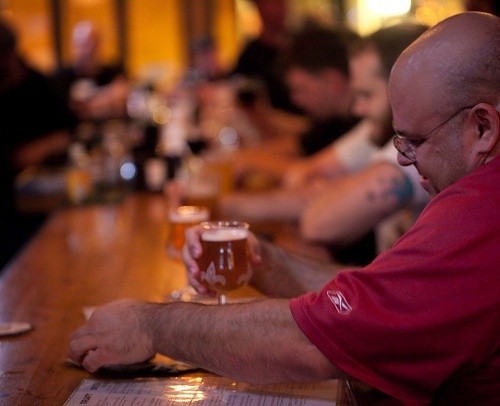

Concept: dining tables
[[0, 194, 349, 406]]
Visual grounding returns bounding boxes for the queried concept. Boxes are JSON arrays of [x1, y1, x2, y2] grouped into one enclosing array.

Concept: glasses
[[393, 106, 475, 162]]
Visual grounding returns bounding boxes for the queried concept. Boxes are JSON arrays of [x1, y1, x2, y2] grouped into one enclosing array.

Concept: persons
[[0, 0, 500, 406]]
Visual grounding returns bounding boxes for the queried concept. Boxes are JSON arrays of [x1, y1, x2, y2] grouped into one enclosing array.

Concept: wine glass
[[166, 205, 208, 301]]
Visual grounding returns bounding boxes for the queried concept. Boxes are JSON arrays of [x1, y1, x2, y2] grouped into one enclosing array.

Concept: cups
[[197, 221, 252, 306], [182, 175, 219, 220]]
